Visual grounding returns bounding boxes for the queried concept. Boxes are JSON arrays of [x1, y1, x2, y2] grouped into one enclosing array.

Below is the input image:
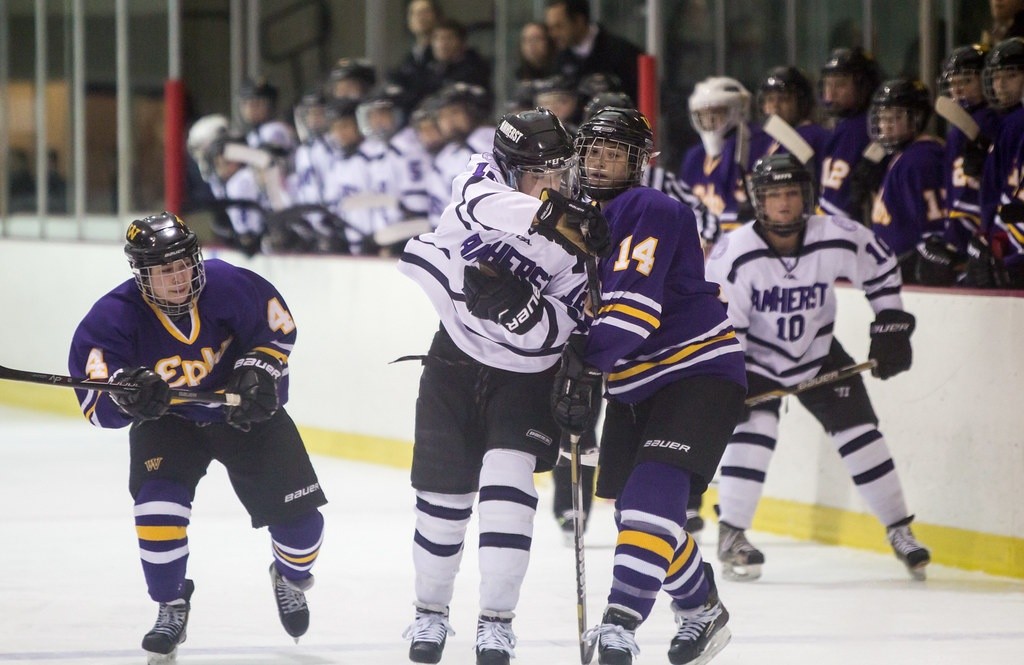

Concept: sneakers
[[142, 578, 195, 665], [269, 560, 315, 644], [476, 609, 516, 665], [886, 513, 931, 583], [598, 607, 639, 665], [552, 444, 600, 535], [717, 519, 766, 583], [669, 562, 733, 664], [410, 599, 450, 665], [685, 508, 702, 534]]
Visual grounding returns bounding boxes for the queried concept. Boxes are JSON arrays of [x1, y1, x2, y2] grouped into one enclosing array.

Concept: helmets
[[572, 106, 655, 201], [530, 71, 634, 109], [688, 75, 753, 162], [491, 105, 580, 176], [937, 38, 1023, 107], [183, 56, 487, 161], [817, 46, 876, 117], [123, 210, 206, 316], [748, 153, 818, 237], [754, 62, 810, 124], [867, 80, 933, 152]]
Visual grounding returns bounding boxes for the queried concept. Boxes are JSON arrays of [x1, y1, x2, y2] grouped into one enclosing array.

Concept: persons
[[550, 106, 748, 665], [184, 0, 1024, 533], [68, 211, 328, 655], [387, 107, 614, 665], [703, 153, 931, 569]]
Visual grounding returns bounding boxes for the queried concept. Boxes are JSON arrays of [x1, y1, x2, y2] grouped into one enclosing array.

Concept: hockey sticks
[[580, 221, 604, 322], [561, 357, 881, 468], [570, 433, 598, 665], [0, 364, 242, 406]]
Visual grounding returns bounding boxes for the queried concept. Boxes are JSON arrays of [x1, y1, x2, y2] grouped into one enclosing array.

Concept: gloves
[[463, 258, 546, 336], [546, 341, 603, 431], [108, 365, 173, 422], [223, 351, 283, 424], [530, 184, 611, 265], [869, 308, 915, 379]]
